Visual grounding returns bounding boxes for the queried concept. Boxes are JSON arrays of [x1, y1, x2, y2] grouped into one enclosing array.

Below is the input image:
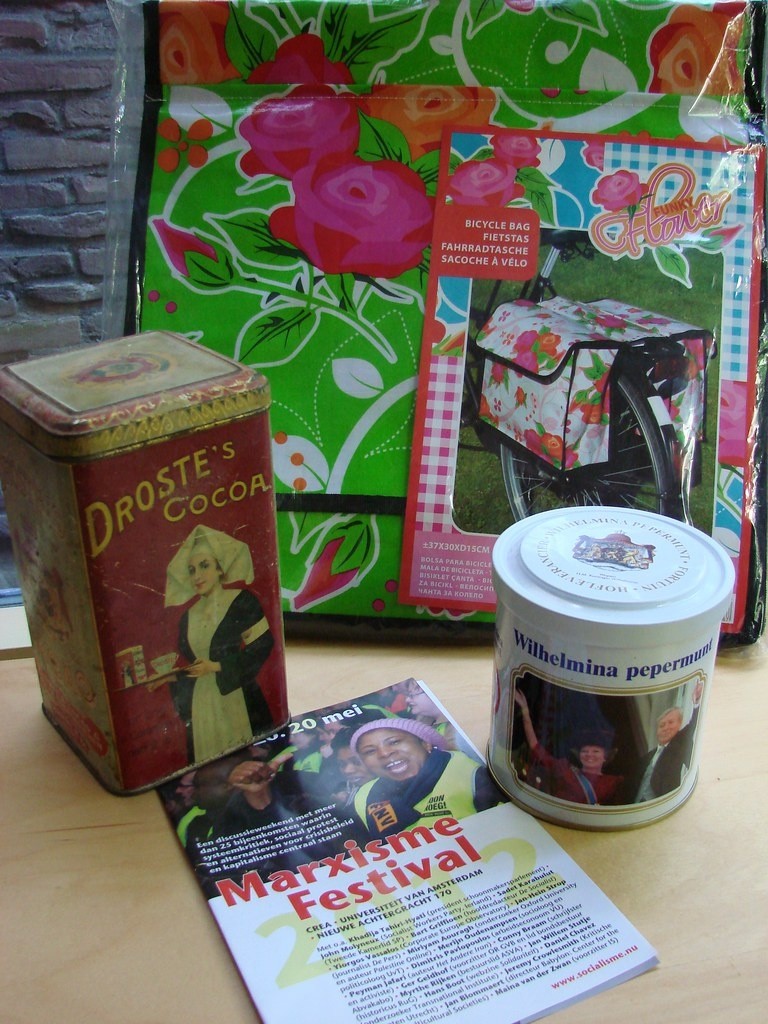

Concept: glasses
[[178, 783, 195, 791], [405, 691, 425, 699]]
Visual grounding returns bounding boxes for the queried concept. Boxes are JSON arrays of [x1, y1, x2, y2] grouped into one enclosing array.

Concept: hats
[[350, 718, 449, 769]]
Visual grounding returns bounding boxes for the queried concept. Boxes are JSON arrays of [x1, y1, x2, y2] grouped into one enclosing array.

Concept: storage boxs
[[0, 330, 293, 801]]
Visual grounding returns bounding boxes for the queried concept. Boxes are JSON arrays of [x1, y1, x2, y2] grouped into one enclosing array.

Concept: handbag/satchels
[[474, 298, 714, 490]]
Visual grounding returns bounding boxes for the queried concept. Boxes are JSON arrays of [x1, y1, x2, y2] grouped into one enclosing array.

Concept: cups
[[490, 508, 736, 834]]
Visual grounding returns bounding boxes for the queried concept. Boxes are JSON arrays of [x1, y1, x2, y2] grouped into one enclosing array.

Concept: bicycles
[[460, 227, 687, 521]]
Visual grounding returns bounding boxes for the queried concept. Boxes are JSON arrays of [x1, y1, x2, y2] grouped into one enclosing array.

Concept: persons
[[147, 524, 276, 761], [516, 689, 630, 806], [163, 676, 505, 894], [121, 661, 134, 688], [625, 679, 701, 803]]
